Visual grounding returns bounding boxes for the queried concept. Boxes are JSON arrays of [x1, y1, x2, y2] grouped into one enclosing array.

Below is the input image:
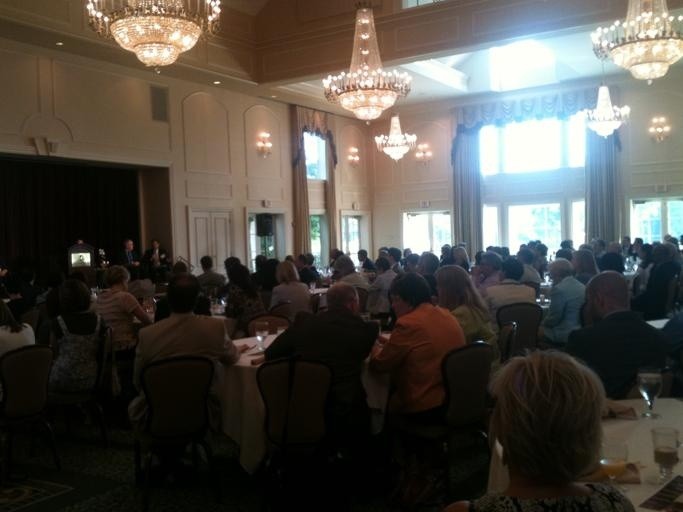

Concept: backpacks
[[48, 314, 104, 392]]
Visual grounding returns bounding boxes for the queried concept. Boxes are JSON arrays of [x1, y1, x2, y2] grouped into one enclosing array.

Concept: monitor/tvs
[[70, 251, 91, 268]]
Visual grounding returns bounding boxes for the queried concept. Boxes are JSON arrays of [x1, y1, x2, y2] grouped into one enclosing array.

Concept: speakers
[[256, 214, 272, 236]]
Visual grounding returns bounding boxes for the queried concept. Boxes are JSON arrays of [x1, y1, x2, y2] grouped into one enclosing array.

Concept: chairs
[[0, 262, 683, 512]]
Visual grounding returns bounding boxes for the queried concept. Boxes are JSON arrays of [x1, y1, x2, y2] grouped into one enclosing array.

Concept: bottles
[[539, 294, 544, 306]]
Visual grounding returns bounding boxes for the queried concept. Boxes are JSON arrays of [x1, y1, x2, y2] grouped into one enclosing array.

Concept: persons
[[536, 258, 588, 351], [441, 349, 637, 512], [373, 272, 464, 459], [326, 240, 548, 317], [48, 280, 109, 359], [2, 300, 34, 357], [567, 270, 670, 402], [262, 282, 371, 445], [127, 272, 249, 455], [153, 272, 212, 326], [167, 253, 323, 340], [661, 281, 681, 400], [97, 265, 153, 353], [486, 257, 536, 330], [435, 266, 501, 370], [555, 235, 681, 319], [1, 235, 167, 321]]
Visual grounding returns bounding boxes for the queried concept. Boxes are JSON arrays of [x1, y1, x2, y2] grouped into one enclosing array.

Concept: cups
[[359, 312, 369, 321], [309, 282, 315, 290], [143, 297, 153, 313]]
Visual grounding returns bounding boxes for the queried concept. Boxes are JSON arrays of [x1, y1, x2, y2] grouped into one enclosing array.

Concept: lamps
[[345, 145, 362, 167], [81, 1, 223, 74], [253, 130, 276, 159], [647, 115, 675, 143], [574, 2, 631, 137], [319, 2, 413, 125], [590, 1, 682, 85], [374, 113, 418, 162], [414, 138, 434, 164]]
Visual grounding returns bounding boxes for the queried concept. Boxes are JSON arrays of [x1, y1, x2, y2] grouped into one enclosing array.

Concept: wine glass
[[598, 438, 629, 485], [635, 365, 662, 418], [253, 320, 269, 353], [543, 272, 550, 284], [648, 426, 680, 486]]
